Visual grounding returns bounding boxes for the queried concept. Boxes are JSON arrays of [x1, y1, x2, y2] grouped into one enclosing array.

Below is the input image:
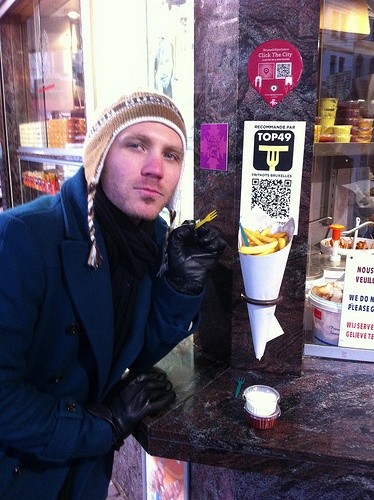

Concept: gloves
[[166, 220, 228, 296], [88, 366, 178, 451]]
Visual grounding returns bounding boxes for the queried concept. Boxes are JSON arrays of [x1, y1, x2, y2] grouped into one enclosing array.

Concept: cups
[[243, 384, 281, 429], [306, 236, 374, 345]]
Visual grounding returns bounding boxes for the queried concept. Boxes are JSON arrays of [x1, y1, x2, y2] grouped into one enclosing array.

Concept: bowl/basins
[[314, 97, 374, 145]]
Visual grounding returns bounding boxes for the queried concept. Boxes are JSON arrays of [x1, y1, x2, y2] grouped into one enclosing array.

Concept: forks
[[195, 210, 218, 230]]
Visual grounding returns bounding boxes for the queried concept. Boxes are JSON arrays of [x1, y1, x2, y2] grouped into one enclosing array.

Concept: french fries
[[238, 228, 288, 255]]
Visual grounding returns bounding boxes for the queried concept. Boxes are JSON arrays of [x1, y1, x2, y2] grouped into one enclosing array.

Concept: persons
[[0, 92, 226, 500], [154, 0, 194, 151]]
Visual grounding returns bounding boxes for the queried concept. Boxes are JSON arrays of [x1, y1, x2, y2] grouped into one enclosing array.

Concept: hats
[[82, 88, 188, 264]]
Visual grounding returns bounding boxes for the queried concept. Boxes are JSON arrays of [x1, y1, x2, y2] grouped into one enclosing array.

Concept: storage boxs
[[19, 117, 87, 150]]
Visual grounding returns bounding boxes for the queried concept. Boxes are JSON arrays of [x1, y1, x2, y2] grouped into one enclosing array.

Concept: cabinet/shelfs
[[303, 0, 374, 363]]
